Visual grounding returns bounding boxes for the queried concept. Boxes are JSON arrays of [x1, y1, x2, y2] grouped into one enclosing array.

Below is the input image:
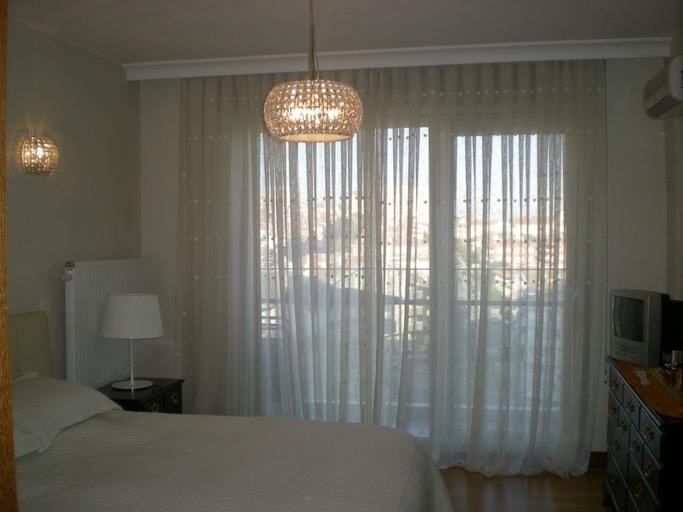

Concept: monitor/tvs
[[609, 289, 683, 369]]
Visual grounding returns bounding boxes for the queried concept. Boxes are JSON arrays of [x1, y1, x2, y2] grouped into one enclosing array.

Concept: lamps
[[19, 128, 57, 174], [260, 1, 364, 146], [98, 290, 164, 393]]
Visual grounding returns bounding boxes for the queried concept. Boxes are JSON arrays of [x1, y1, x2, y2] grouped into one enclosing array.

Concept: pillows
[[11, 371, 123, 458]]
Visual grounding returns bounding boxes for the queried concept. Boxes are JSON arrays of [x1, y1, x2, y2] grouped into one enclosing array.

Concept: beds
[[9, 308, 458, 512]]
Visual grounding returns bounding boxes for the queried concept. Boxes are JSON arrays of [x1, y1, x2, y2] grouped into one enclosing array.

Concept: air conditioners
[[640, 55, 682, 120]]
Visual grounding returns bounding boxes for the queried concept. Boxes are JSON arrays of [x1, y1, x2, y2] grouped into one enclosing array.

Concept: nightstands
[[99, 375, 188, 414]]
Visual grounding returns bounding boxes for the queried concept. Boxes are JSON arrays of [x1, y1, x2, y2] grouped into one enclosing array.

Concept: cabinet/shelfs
[[600, 354, 683, 511]]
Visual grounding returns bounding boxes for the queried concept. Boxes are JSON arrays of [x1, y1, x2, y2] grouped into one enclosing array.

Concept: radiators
[[60, 256, 153, 390]]
